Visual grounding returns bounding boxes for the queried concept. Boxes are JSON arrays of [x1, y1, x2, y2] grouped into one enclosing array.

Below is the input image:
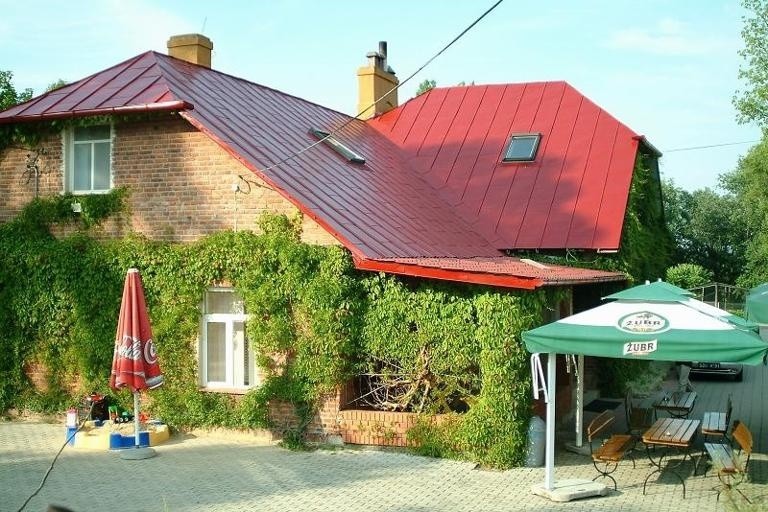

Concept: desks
[[651, 391, 697, 420], [641, 417, 701, 500]]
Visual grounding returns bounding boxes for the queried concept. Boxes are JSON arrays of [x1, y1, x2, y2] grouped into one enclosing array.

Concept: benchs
[[587, 409, 637, 491], [702, 393, 733, 456], [704, 420, 754, 504], [625, 386, 657, 454]]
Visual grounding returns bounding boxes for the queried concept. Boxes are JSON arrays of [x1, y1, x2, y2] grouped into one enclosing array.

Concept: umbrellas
[[107, 267, 162, 448]]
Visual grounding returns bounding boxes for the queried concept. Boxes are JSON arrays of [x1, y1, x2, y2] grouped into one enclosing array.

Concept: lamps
[[71, 203, 82, 213]]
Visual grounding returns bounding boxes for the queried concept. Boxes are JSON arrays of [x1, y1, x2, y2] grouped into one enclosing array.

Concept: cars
[[673, 361, 744, 383]]
